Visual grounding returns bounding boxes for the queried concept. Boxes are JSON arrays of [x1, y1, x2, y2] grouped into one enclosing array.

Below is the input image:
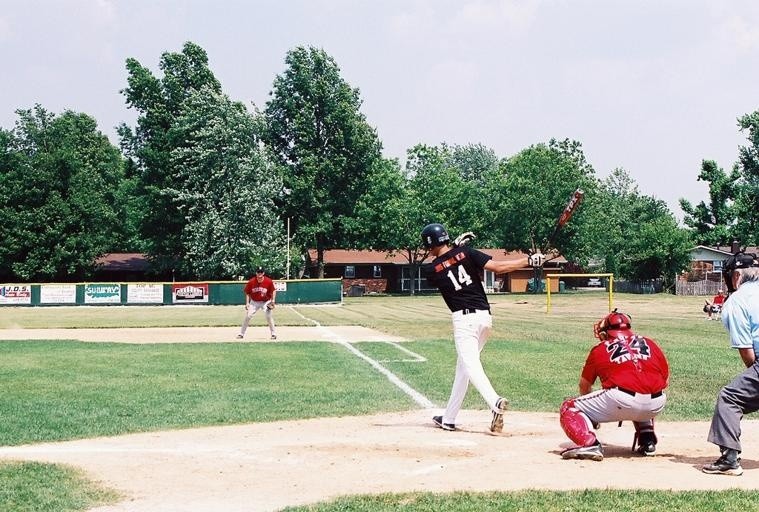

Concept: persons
[[234, 266, 278, 340], [701, 250, 759, 477], [421, 223, 547, 437], [703, 290, 725, 321], [558, 307, 670, 462]]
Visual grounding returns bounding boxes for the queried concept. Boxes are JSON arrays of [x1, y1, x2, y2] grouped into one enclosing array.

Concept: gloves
[[528, 252, 546, 268], [453, 231, 476, 246]]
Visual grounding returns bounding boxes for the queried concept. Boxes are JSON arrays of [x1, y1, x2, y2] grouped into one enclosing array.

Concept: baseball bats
[[542, 189, 583, 255]]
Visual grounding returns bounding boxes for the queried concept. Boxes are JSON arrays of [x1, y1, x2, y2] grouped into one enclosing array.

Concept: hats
[[256, 266, 264, 272]]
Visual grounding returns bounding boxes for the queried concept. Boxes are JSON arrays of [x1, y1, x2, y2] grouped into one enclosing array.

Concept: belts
[[464, 307, 492, 315], [614, 385, 662, 400]]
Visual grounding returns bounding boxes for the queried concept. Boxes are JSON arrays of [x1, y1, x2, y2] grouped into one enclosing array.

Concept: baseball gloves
[[267, 302, 275, 310]]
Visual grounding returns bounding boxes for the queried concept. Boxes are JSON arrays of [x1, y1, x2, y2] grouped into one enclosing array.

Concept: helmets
[[594, 308, 634, 338], [722, 249, 758, 291], [420, 224, 449, 246]]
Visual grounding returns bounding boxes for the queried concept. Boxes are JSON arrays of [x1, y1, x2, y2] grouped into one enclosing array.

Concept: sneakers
[[562, 443, 604, 462], [432, 415, 455, 430], [490, 397, 508, 434], [703, 455, 743, 475], [637, 440, 657, 453]]
